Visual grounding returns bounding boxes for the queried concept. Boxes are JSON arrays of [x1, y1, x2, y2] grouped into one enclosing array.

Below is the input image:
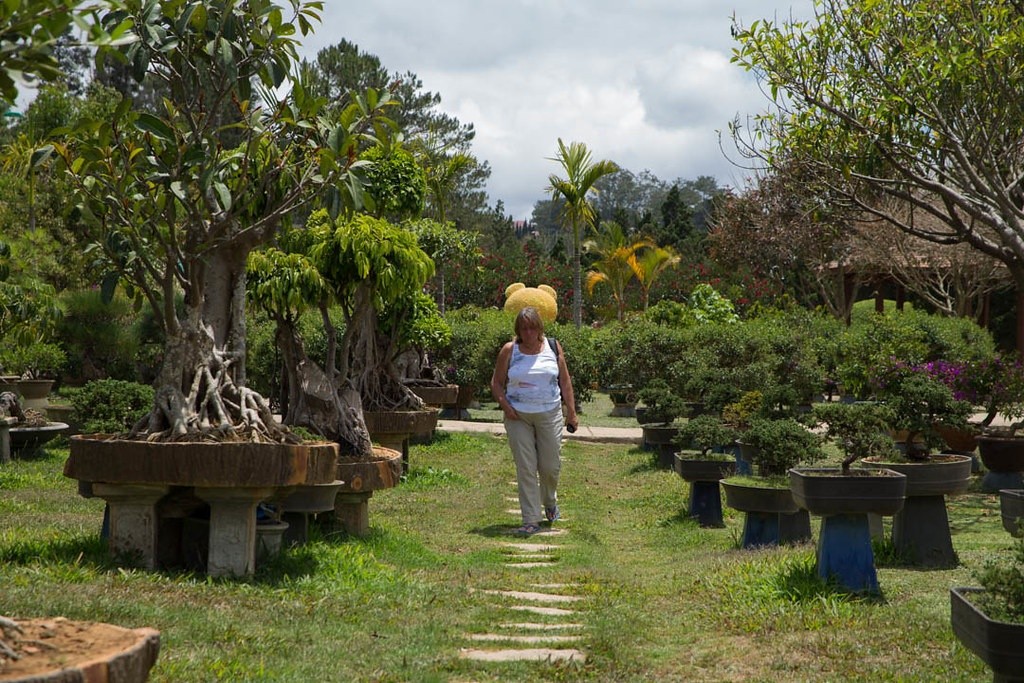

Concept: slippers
[[515, 523, 541, 535], [544, 506, 560, 523]]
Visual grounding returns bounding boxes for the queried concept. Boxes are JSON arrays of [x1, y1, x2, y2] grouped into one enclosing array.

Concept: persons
[[491, 306, 579, 533]]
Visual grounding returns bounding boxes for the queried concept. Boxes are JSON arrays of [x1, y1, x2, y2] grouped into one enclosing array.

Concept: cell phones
[[566, 424, 575, 433]]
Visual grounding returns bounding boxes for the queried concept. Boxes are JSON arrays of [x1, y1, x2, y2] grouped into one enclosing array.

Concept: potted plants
[[950, 517, 1024, 683], [592, 306, 1023, 605]]
